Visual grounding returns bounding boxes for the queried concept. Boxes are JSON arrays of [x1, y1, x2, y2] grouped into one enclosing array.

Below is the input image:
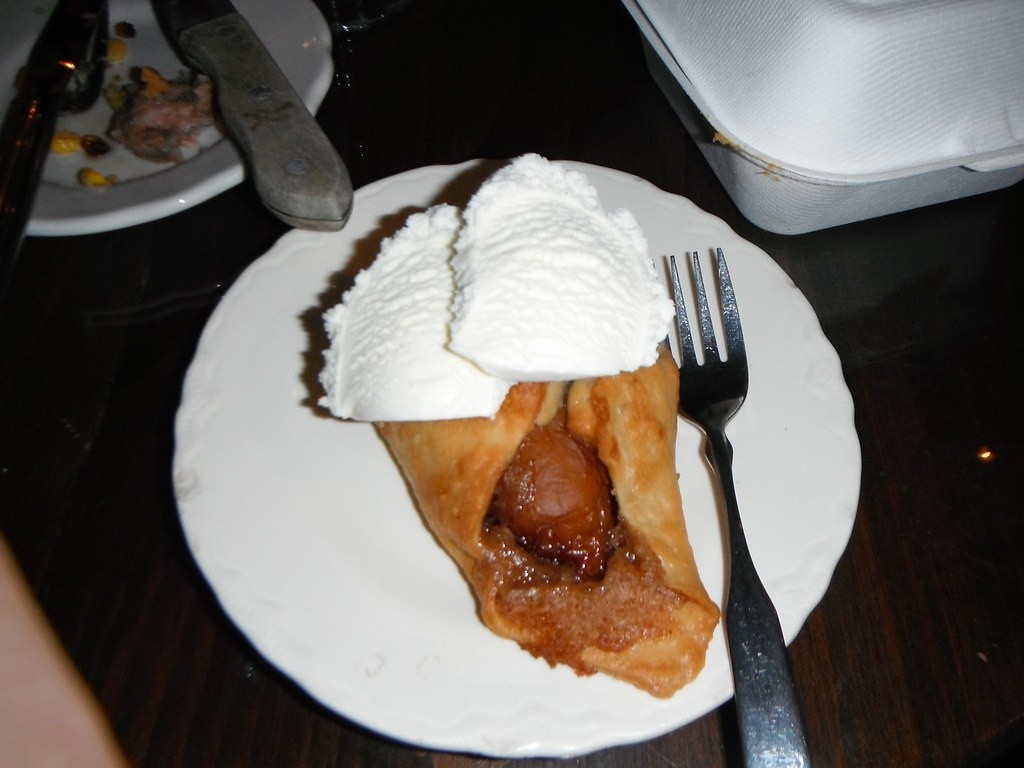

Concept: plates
[[0, 0, 335, 237], [170, 160, 862, 757]]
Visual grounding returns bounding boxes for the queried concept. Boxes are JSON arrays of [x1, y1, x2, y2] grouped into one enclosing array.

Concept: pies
[[373, 335, 718, 700]]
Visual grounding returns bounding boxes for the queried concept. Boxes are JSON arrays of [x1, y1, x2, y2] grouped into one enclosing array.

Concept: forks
[[664, 248, 815, 768]]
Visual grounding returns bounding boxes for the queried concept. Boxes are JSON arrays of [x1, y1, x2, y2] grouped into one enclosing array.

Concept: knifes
[[150, 0, 354, 232]]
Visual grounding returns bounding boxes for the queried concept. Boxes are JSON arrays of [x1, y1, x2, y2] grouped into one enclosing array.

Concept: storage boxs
[[622, 0, 1024, 236]]
